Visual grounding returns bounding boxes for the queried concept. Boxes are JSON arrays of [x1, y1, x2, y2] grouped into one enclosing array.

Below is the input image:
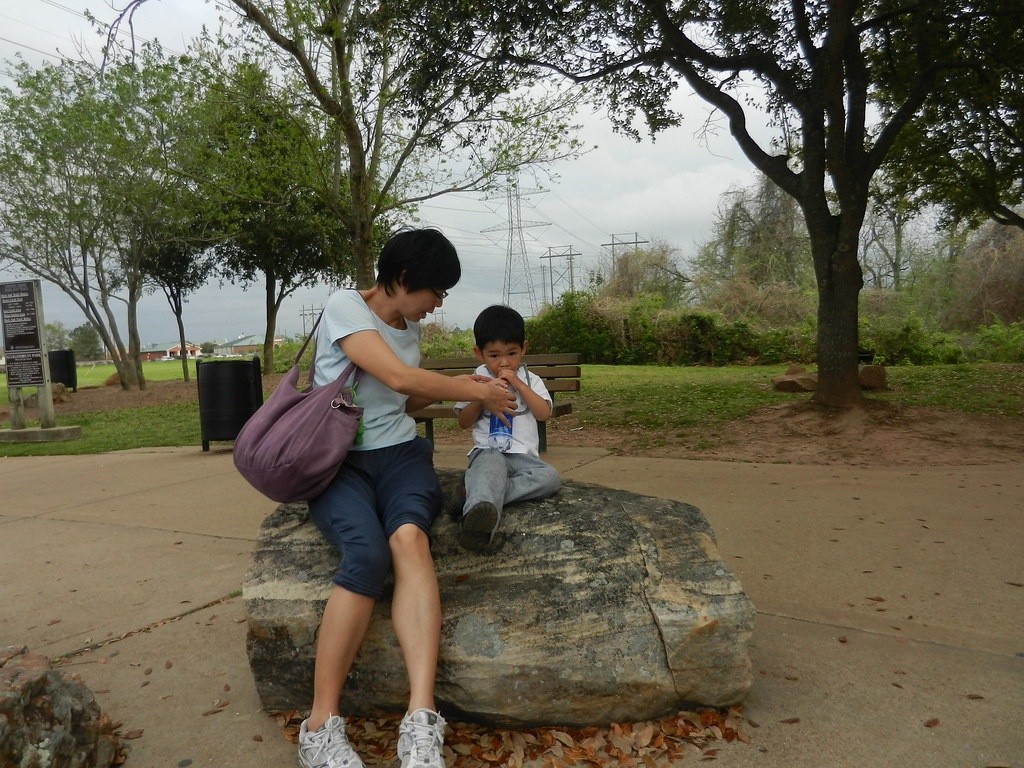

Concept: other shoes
[[457, 501, 498, 553]]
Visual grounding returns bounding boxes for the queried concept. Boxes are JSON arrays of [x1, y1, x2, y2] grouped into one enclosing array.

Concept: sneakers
[[297, 712, 366, 768], [398, 708, 447, 768]]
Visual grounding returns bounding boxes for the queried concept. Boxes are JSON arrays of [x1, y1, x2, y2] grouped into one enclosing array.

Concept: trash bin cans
[[195, 357, 263, 452], [48, 349, 77, 391]]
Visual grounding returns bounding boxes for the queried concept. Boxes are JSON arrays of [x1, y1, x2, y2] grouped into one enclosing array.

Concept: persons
[[451, 305, 563, 554], [296, 224, 518, 768]]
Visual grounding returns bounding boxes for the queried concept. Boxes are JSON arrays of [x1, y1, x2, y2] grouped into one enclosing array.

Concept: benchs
[[408, 353, 581, 451]]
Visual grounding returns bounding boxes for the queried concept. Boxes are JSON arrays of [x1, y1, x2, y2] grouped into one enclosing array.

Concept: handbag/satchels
[[233, 288, 374, 503]]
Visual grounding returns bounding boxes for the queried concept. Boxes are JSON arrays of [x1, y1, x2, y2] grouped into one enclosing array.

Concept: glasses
[[427, 282, 449, 303]]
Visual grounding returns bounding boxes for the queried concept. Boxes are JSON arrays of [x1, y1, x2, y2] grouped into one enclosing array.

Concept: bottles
[[488, 383, 515, 451]]
